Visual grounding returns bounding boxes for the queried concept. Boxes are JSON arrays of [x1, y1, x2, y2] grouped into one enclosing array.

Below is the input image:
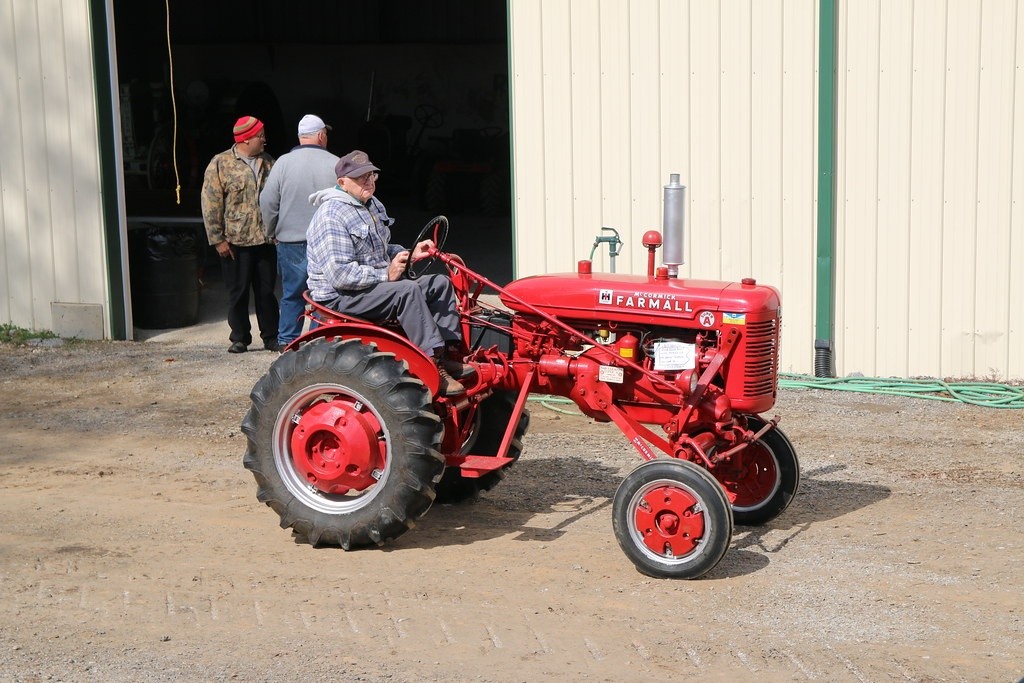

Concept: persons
[[305, 150, 482, 398], [201, 112, 341, 353]]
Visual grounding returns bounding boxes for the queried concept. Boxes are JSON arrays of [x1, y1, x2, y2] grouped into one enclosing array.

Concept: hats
[[233, 116, 264, 143], [335, 150, 381, 178], [298, 114, 331, 136]]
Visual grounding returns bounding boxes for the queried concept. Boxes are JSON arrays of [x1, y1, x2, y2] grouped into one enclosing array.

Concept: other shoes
[[228, 341, 247, 353], [447, 361, 475, 378], [433, 365, 466, 396], [265, 343, 279, 350]]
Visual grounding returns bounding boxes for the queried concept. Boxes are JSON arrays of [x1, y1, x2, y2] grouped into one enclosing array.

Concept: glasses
[[253, 133, 265, 140], [355, 173, 379, 184]]
